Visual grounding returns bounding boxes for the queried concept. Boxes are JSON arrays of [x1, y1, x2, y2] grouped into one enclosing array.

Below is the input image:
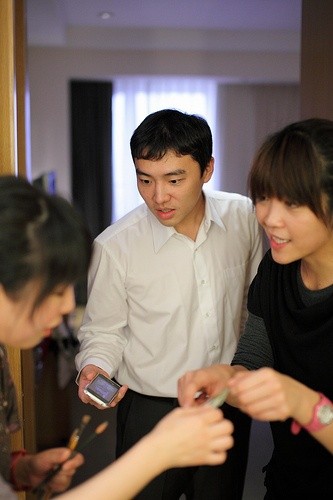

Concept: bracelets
[[10, 450, 33, 493]]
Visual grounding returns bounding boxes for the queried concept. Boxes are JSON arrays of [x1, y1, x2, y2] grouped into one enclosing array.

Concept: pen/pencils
[[31, 421, 108, 495], [41, 415, 91, 499]]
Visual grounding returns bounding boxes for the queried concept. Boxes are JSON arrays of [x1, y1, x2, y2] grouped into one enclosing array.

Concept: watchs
[[291, 391, 333, 434]]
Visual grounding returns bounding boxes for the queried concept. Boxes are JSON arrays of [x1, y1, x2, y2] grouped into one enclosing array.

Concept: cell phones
[[84, 372, 121, 408]]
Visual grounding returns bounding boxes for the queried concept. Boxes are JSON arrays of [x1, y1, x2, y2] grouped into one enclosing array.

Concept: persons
[[177, 118, 333, 500], [0, 174, 233, 500], [75, 109, 271, 500]]
